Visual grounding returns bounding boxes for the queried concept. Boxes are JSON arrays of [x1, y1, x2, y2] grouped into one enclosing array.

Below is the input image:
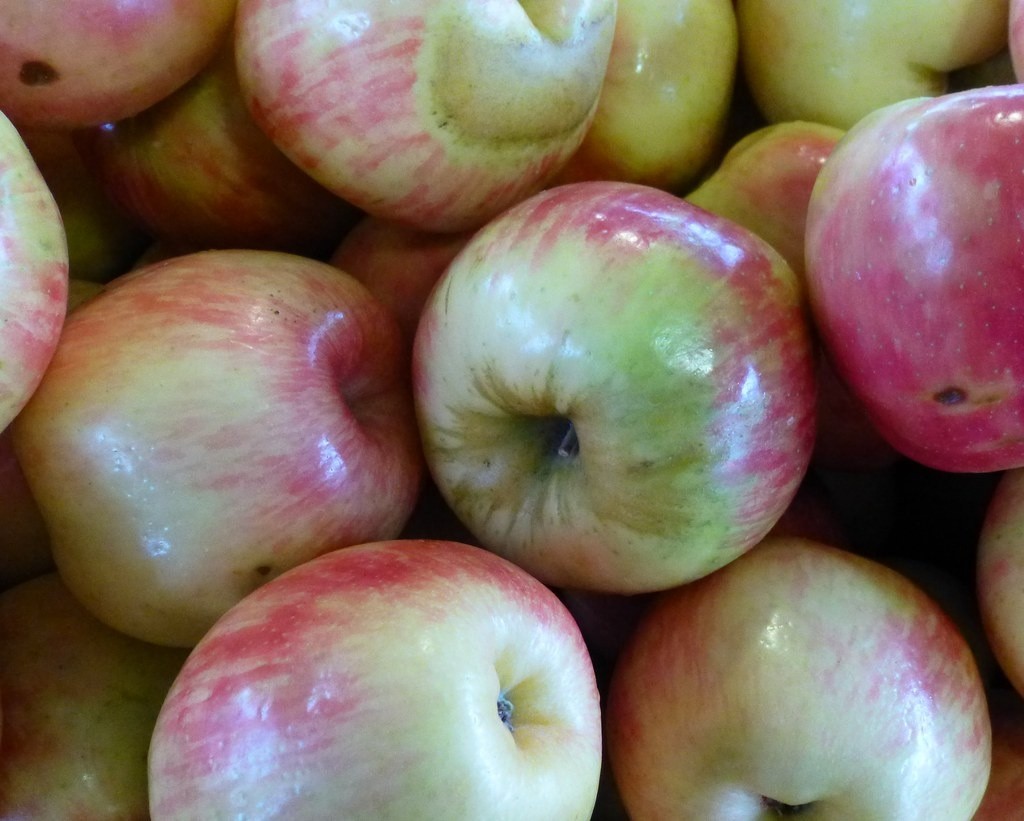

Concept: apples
[[0, 0, 1024, 821]]
